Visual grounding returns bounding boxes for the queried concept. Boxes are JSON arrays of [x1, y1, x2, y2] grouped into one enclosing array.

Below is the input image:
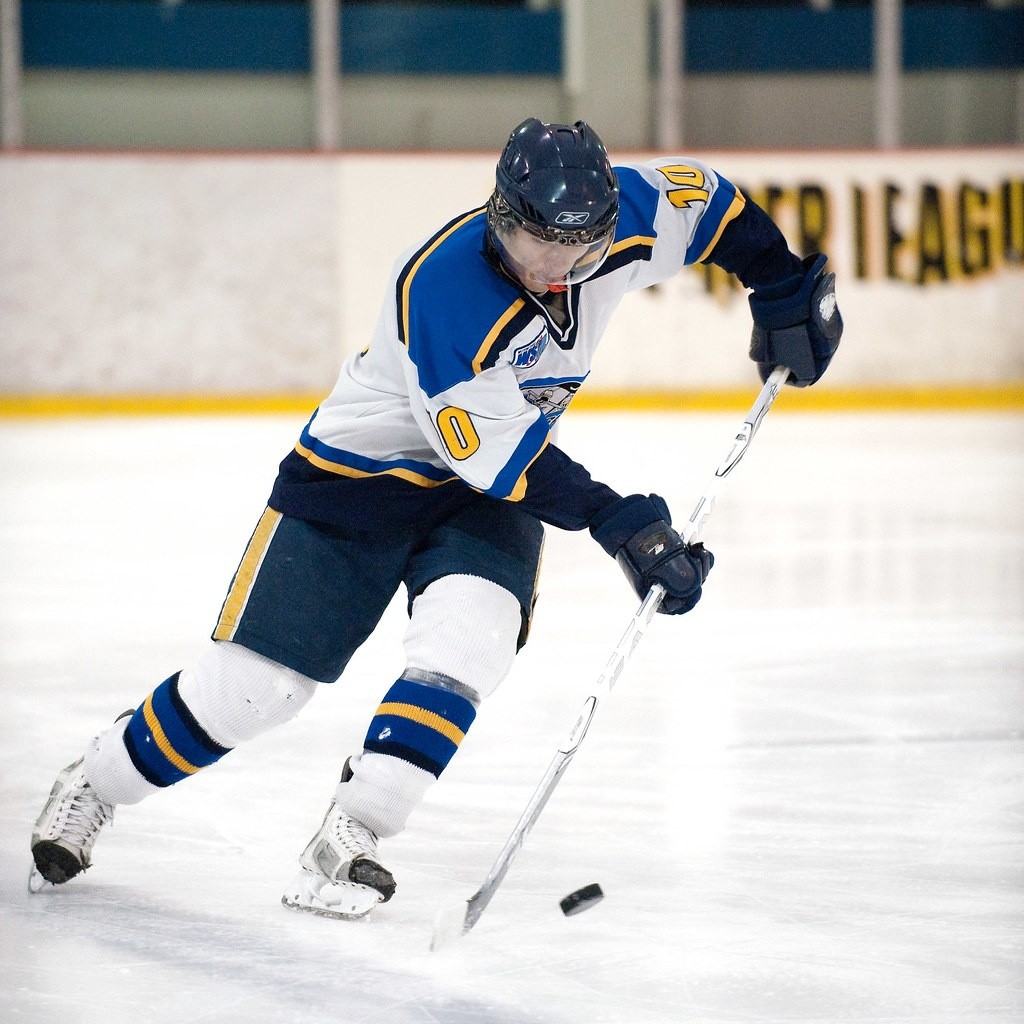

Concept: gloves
[[747, 254, 843, 387], [590, 493, 716, 615]]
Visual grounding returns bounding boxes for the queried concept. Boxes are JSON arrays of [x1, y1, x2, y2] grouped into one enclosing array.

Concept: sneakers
[[283, 756, 394, 921], [28, 708, 139, 892]]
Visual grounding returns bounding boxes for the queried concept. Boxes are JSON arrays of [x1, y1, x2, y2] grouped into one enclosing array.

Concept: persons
[[27, 119, 844, 923]]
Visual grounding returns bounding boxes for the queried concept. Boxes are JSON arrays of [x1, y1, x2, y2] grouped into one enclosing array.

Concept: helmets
[[488, 117, 617, 244]]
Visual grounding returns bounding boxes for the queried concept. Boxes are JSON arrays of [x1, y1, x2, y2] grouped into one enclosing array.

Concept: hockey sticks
[[423, 362, 795, 957]]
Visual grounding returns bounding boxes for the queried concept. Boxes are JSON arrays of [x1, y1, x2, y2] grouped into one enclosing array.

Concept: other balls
[[558, 881, 605, 920]]
